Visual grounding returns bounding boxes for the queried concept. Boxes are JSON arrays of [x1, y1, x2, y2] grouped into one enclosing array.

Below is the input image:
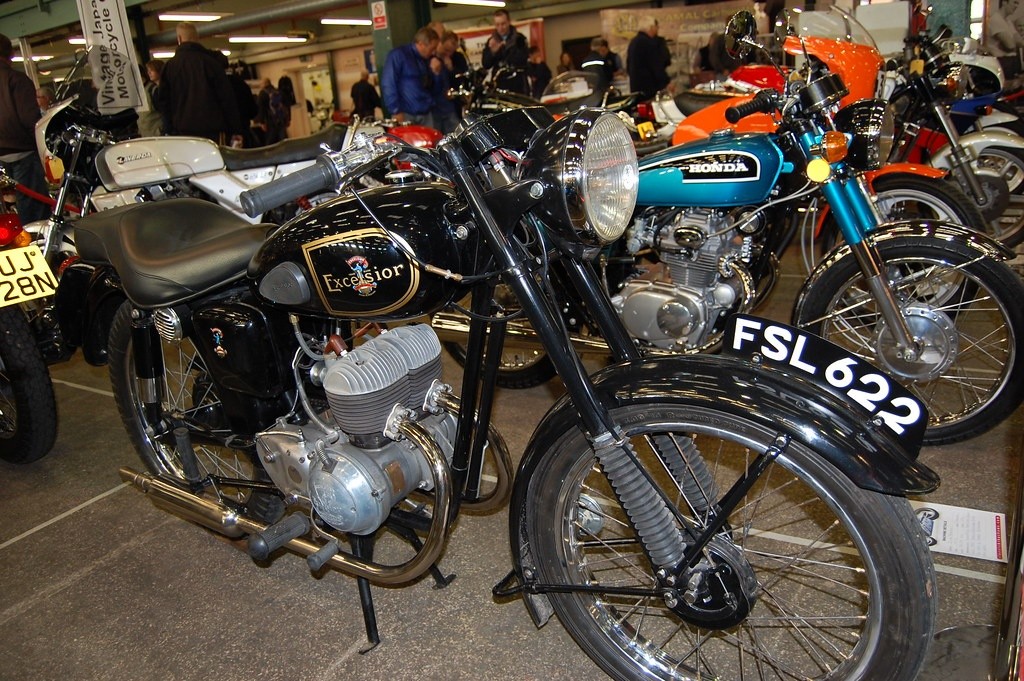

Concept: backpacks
[[264, 88, 280, 111]]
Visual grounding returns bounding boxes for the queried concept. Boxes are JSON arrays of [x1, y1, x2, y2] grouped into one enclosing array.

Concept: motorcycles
[[0, 3, 1024, 681]]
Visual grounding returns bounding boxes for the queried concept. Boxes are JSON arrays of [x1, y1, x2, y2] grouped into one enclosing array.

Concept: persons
[[36, 87, 54, 117], [157, 22, 229, 139], [133, 59, 165, 138], [987, 0, 1024, 75], [481, 8, 530, 96], [380, 22, 468, 133], [557, 52, 571, 75], [695, 15, 734, 82], [527, 45, 553, 99], [257, 78, 291, 146], [582, 38, 622, 89], [351, 69, 382, 122], [0, 34, 51, 225], [626, 15, 676, 103]]
